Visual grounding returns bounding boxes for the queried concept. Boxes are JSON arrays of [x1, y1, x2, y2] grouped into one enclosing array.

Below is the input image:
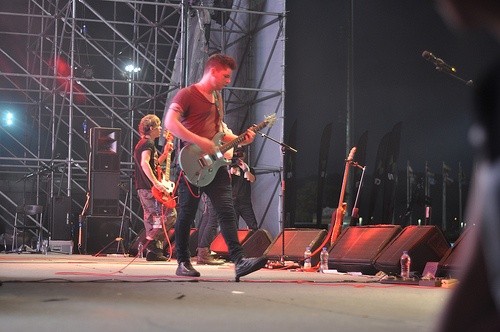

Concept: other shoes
[[146, 251, 168, 261], [144, 241, 164, 256]]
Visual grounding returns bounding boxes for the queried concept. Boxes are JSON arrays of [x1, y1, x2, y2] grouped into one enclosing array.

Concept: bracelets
[[243, 168, 248, 172]]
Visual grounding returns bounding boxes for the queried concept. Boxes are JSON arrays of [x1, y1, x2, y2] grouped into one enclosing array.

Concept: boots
[[195, 247, 225, 265]]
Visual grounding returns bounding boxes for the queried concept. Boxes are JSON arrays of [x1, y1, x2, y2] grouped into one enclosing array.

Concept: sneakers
[[235, 254, 269, 278], [176, 260, 200, 276]]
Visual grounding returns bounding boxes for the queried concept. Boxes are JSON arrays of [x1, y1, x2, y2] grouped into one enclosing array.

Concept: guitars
[[151, 130, 176, 209], [178, 113, 277, 188]]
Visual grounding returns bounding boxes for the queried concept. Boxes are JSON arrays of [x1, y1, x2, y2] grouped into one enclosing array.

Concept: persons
[[432, 62, 500, 332], [135, 114, 174, 256], [164, 55, 269, 277], [197, 193, 226, 265], [227, 147, 257, 230]]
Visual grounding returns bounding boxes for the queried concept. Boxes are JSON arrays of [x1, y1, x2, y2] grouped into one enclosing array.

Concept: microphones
[[421, 51, 456, 73]]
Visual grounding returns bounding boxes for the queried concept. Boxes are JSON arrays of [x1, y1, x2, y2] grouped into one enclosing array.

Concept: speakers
[[209, 227, 271, 260], [372, 225, 451, 276], [324, 225, 403, 274], [127, 228, 175, 256], [165, 228, 198, 257], [435, 224, 478, 278], [87, 127, 121, 215], [84, 215, 129, 254], [263, 227, 328, 267]]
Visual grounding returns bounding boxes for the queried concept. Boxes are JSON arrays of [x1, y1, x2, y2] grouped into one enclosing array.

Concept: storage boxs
[[36, 240, 75, 255]]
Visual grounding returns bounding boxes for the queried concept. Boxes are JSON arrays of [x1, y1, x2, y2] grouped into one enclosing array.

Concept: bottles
[[400, 250, 410, 278], [320, 247, 329, 272], [304, 246, 312, 268], [138, 242, 143, 258], [42, 242, 47, 255]]
